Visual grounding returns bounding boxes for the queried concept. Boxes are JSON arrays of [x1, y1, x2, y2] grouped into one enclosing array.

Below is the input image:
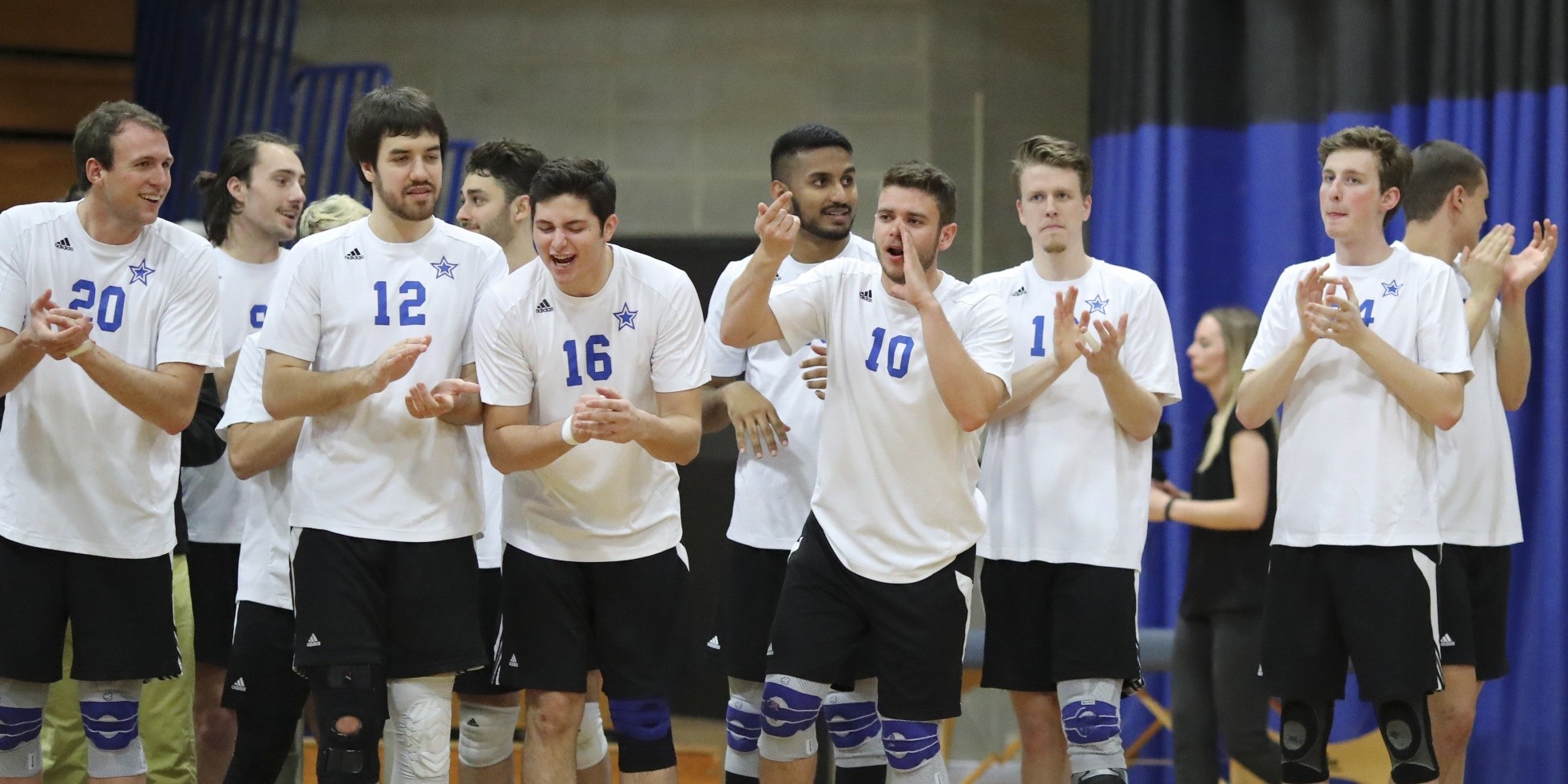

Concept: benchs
[[940, 623, 1178, 784]]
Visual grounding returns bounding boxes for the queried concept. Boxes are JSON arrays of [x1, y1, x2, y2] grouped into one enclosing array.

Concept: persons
[[967, 135, 1186, 783], [258, 85, 509, 784], [297, 193, 374, 238], [704, 125, 890, 784], [473, 157, 710, 783], [723, 160, 1014, 784], [0, 99, 224, 783], [216, 330, 310, 783], [192, 130, 306, 783], [1234, 125, 1474, 784], [458, 145, 614, 783], [1145, 306, 1285, 784], [1400, 142, 1557, 784]]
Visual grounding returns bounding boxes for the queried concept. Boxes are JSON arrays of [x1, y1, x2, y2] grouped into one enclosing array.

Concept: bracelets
[[1164, 496, 1180, 521], [63, 339, 97, 358], [561, 415, 580, 447]]
